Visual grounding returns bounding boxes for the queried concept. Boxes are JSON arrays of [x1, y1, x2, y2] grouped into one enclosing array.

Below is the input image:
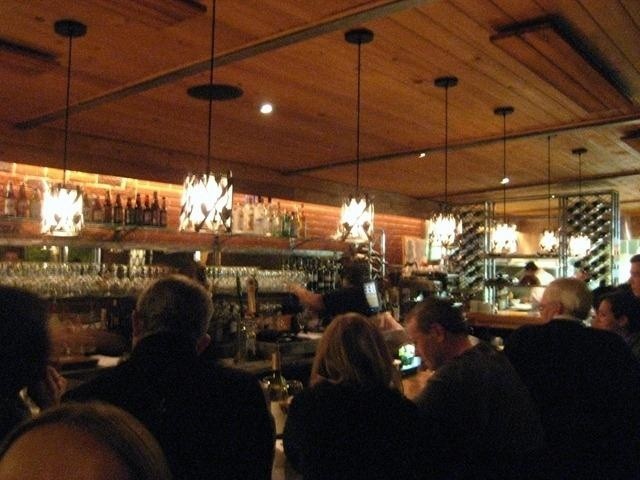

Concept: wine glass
[[259, 270, 305, 293], [1, 262, 245, 298]]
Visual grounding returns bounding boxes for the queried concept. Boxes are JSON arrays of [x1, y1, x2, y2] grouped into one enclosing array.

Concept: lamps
[[492, 102, 516, 255], [540, 131, 558, 256], [335, 27, 376, 246], [178, 82, 243, 233], [425, 73, 462, 253], [39, 16, 89, 240], [566, 146, 591, 260]]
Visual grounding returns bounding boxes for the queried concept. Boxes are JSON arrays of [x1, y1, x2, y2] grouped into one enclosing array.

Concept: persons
[[1, 247, 23, 261], [509, 256, 640, 365], [0, 397, 174, 480], [283, 312, 418, 480], [505, 278, 639, 479], [0, 283, 69, 441], [59, 274, 277, 480], [282, 262, 376, 321], [76, 251, 219, 365], [404, 297, 555, 479]]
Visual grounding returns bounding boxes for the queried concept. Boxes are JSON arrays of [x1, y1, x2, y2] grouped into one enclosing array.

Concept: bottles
[[267, 351, 288, 439], [245, 320, 257, 362], [1, 184, 169, 227], [233, 196, 305, 236], [305, 259, 336, 291], [246, 271, 259, 317], [207, 303, 244, 343], [279, 257, 304, 270]]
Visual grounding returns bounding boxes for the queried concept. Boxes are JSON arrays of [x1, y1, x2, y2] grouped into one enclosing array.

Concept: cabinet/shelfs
[[1, 212, 374, 361], [483, 252, 564, 312]]
